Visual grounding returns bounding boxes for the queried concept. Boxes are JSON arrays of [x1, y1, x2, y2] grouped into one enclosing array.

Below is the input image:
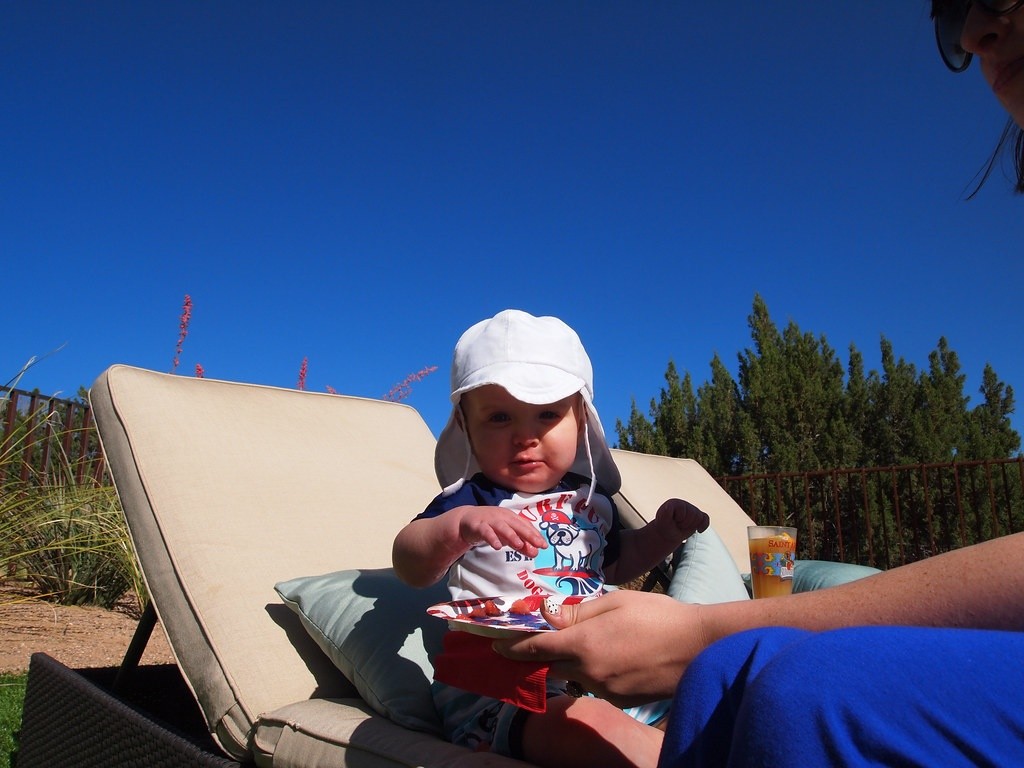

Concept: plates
[[427, 595, 603, 638]]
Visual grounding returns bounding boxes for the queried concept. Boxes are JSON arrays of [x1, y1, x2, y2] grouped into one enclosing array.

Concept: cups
[[747, 525, 797, 599]]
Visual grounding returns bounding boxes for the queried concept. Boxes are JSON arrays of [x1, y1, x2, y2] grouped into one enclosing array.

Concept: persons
[[492, 0, 1024, 767], [391, 307, 712, 768]]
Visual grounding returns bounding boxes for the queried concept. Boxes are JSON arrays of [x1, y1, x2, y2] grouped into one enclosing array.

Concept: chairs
[[17, 363, 542, 768], [601, 446, 761, 596]]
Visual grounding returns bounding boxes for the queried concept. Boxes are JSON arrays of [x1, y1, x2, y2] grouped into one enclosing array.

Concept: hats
[[435, 308, 622, 497]]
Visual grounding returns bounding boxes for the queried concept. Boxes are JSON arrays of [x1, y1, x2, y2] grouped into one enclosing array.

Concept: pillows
[[273, 565, 453, 735], [666, 526, 751, 603], [741, 558, 887, 594]]
